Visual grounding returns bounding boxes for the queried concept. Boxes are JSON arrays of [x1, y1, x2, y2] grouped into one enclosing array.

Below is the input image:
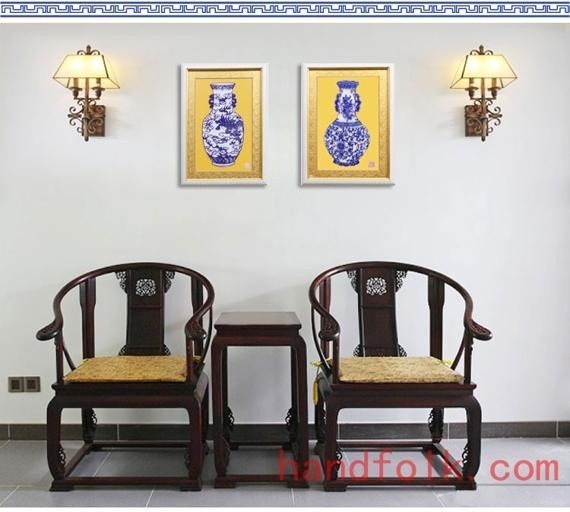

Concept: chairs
[[37, 262, 215, 493], [308, 261, 492, 491]]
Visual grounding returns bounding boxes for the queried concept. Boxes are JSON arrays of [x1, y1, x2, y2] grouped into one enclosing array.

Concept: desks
[[210, 312, 307, 488]]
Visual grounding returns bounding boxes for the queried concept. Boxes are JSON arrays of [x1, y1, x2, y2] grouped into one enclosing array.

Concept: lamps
[[447, 45, 518, 143], [52, 43, 121, 140]]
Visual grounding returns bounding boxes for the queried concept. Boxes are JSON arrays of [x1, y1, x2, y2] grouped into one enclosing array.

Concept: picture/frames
[[177, 61, 396, 186]]
